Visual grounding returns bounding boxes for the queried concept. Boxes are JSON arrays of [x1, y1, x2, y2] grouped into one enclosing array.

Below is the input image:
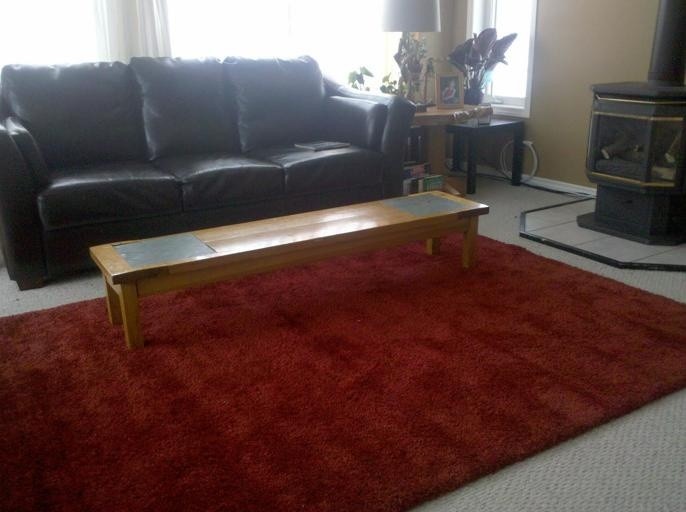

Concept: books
[[404, 132, 444, 193], [292, 138, 351, 153]]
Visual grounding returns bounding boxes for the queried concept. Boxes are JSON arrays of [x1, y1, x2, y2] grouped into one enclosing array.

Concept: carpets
[[0, 227, 686, 511]]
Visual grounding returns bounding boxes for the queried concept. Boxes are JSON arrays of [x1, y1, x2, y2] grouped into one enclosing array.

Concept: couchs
[[1, 58, 417, 291]]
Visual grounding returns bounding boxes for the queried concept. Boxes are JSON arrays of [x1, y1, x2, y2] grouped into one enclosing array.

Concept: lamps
[[383, 0, 440, 35]]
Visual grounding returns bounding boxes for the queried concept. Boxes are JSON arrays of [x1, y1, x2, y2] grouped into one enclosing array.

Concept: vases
[[465, 79, 484, 106]]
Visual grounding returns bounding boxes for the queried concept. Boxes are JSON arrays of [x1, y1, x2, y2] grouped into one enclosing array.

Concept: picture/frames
[[434, 72, 465, 110]]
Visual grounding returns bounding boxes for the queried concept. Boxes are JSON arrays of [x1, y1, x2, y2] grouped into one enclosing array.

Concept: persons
[[441, 78, 456, 103]]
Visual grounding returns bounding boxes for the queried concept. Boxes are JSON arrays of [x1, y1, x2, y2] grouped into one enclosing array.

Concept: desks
[[411, 103, 525, 195]]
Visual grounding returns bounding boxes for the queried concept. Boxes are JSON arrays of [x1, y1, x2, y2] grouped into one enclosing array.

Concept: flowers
[[449, 28, 518, 88]]
